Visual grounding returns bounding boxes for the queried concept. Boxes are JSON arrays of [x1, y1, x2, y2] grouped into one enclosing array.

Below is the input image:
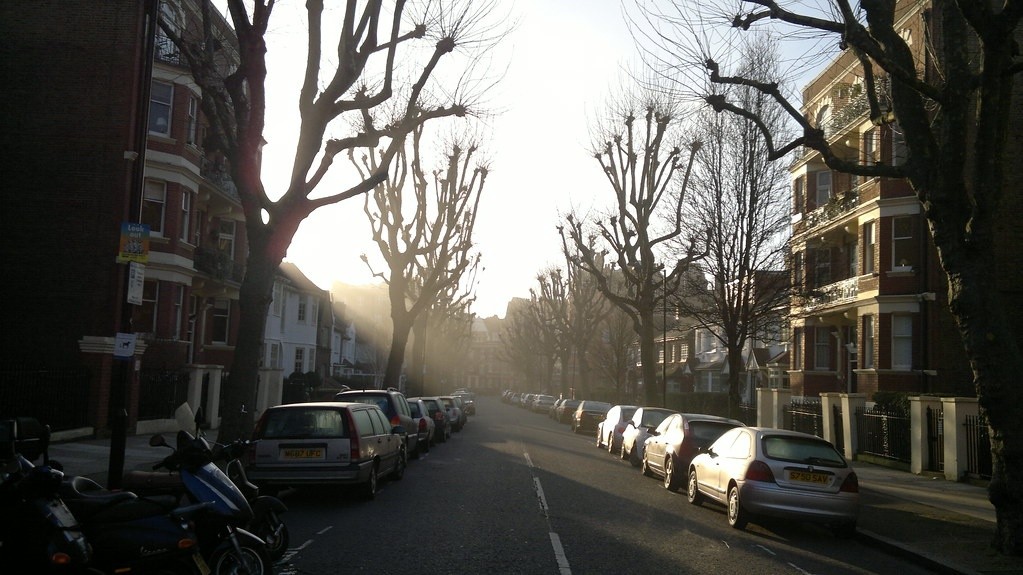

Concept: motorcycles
[[6, 402, 273, 575], [206, 425, 293, 561]]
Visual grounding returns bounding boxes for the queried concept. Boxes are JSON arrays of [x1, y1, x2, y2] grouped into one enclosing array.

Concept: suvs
[[334, 385, 420, 469]]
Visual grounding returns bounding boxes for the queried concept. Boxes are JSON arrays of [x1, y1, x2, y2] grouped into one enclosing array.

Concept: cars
[[247, 402, 406, 501], [420, 397, 451, 442], [448, 386, 478, 420], [498, 389, 579, 424], [439, 396, 466, 432], [408, 398, 436, 452], [643, 411, 751, 491], [621, 408, 678, 467], [571, 400, 614, 434], [687, 427, 863, 528], [597, 404, 640, 454]]
